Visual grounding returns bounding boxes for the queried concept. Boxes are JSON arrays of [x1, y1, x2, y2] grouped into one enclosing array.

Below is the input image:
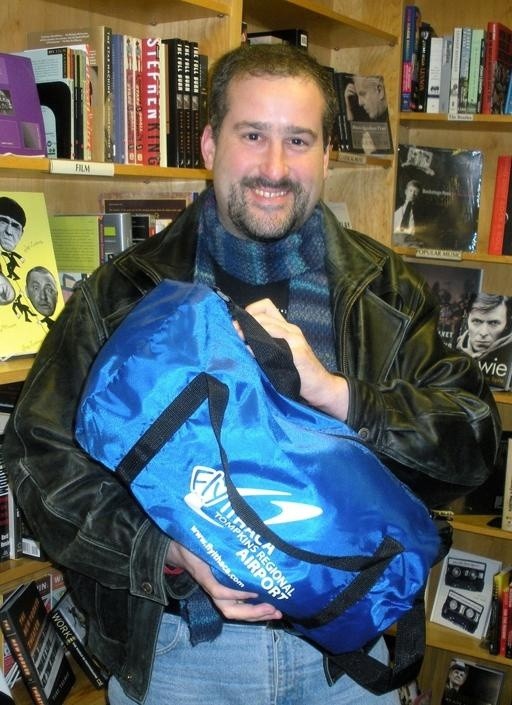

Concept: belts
[[152, 587, 283, 628]]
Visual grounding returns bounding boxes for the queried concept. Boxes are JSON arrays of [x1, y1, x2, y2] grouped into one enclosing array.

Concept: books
[[407, 261, 512, 394], [2, 574, 108, 699], [397, 8, 511, 112], [1, 191, 201, 360], [0, 395, 44, 562], [391, 134, 510, 255], [243, 28, 392, 166], [441, 655, 507, 699], [429, 542, 511, 659], [1, 24, 213, 170]]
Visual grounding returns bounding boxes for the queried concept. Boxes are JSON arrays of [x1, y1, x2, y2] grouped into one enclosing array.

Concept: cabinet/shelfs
[[391, 0, 512, 703], [0, 0, 401, 703]]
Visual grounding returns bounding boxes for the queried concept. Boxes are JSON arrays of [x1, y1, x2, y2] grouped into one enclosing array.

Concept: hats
[[0, 196, 27, 227], [450, 660, 466, 671]]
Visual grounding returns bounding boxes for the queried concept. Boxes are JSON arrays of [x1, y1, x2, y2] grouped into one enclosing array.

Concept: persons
[[0, 40, 501, 699], [27, 267, 58, 316], [395, 181, 424, 232], [460, 292, 511, 359], [450, 661, 469, 691], [0, 198, 26, 254], [344, 72, 386, 124]]
